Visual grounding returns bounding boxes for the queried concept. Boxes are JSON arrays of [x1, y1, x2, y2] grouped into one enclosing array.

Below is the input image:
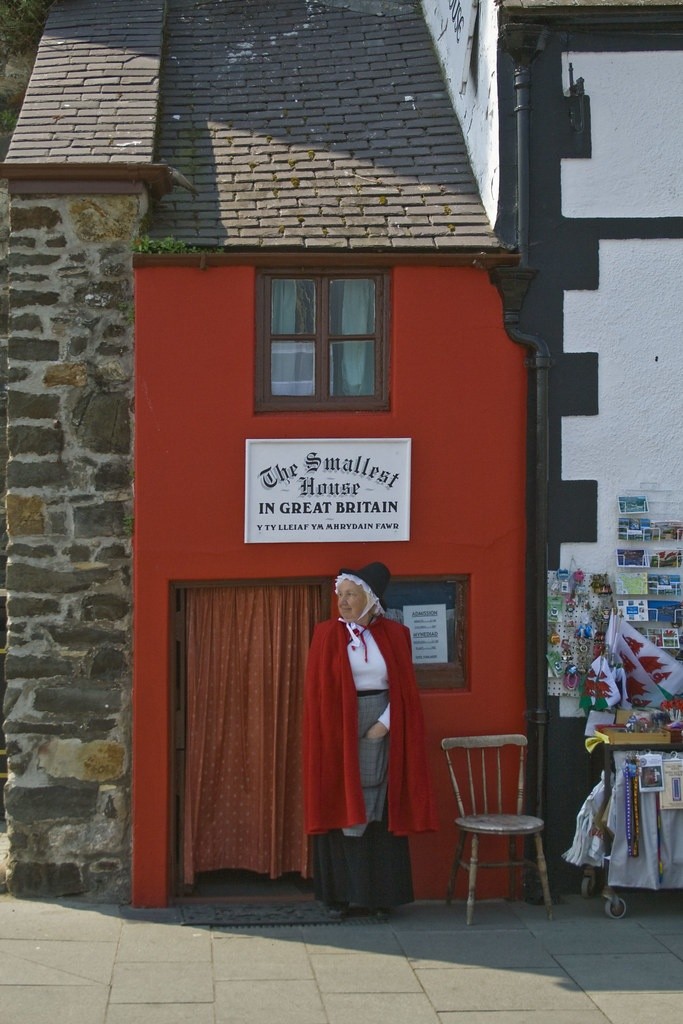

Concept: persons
[[301, 559, 440, 921]]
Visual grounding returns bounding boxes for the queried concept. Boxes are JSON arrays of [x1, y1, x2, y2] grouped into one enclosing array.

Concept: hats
[[338, 561, 392, 612]]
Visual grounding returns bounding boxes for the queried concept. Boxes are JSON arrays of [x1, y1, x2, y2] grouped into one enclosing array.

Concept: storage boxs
[[594, 723, 671, 745]]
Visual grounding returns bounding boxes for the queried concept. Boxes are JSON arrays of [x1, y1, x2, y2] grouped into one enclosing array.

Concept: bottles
[[628, 715, 638, 733], [639, 718, 649, 734]]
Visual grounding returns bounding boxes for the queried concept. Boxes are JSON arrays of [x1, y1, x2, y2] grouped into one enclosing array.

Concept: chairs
[[440, 734, 553, 929]]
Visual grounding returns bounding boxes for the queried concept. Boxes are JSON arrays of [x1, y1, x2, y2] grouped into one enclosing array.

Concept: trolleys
[[579, 740, 683, 918]]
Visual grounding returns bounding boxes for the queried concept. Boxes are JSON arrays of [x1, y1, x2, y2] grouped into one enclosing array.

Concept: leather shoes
[[370, 906, 391, 920], [327, 903, 344, 919]]
